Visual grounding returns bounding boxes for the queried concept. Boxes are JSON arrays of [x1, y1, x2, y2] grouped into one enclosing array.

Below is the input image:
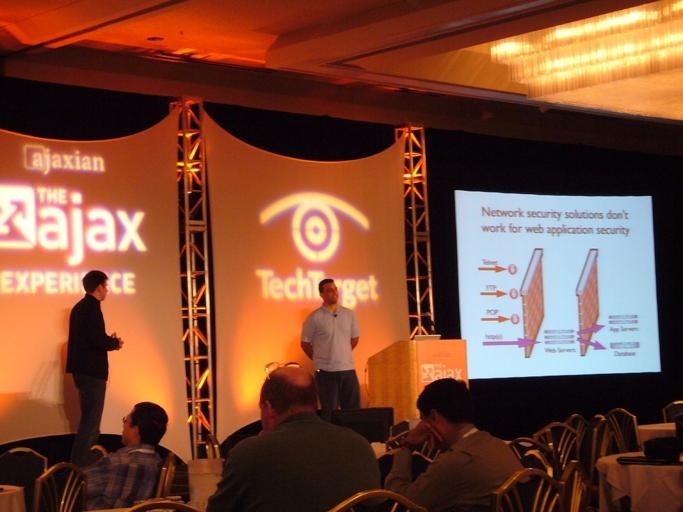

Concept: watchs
[[402, 439, 416, 451]]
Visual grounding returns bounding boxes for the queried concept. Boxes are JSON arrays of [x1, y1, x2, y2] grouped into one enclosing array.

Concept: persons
[[82, 401, 169, 511], [206, 361, 379, 512], [384, 379, 536, 512], [65, 271, 124, 460], [302, 278, 360, 412]]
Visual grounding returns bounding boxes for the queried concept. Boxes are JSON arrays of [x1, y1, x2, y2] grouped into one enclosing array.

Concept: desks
[[367, 339, 468, 431], [1, 482, 25, 512], [597, 450, 683, 511], [637, 422, 680, 450]]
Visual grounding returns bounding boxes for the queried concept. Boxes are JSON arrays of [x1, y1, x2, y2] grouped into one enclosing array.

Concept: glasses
[[264, 361, 304, 381], [122, 416, 127, 423]]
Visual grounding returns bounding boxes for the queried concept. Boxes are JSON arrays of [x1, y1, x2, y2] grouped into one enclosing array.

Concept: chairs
[[126, 499, 200, 512], [508, 436, 561, 482], [33, 462, 90, 512], [7, 447, 49, 477], [591, 414, 607, 432], [88, 443, 106, 461], [661, 400, 682, 422], [489, 467, 567, 512], [329, 487, 426, 511], [567, 413, 586, 427], [571, 425, 606, 511], [541, 422, 576, 461], [155, 452, 175, 498], [533, 429, 552, 446], [605, 407, 642, 454]]
